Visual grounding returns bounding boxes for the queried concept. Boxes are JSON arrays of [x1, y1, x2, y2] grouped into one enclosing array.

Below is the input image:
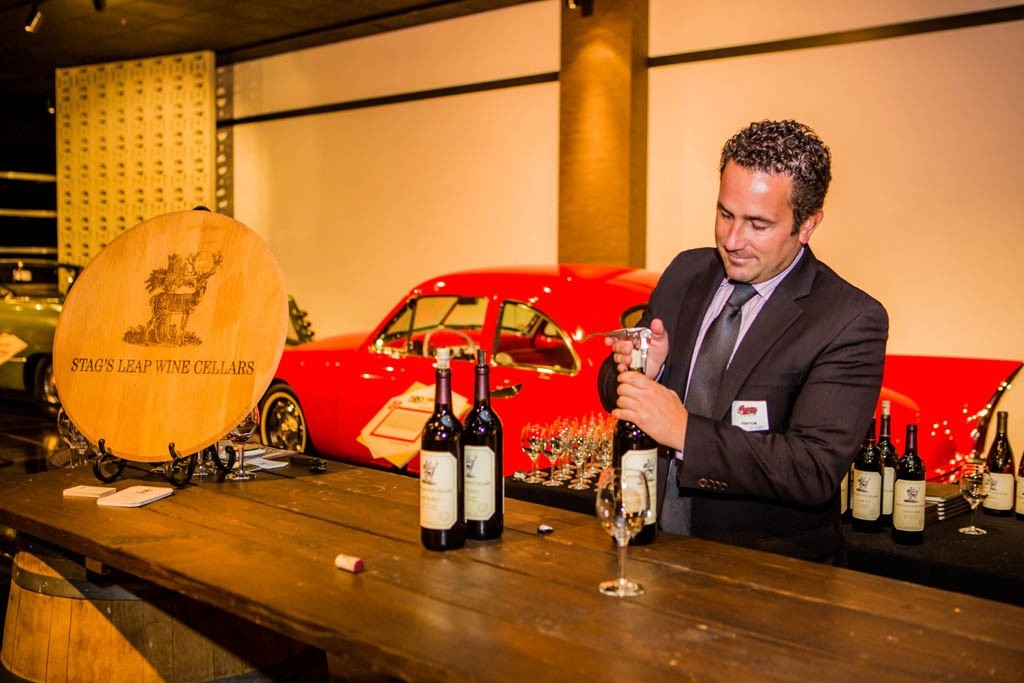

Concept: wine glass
[[193, 403, 260, 480], [58, 406, 101, 468], [595, 467, 651, 597], [958, 464, 991, 535], [520, 413, 615, 490]]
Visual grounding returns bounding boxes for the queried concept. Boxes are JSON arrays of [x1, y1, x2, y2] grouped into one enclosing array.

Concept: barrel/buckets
[[0, 535, 328, 683]]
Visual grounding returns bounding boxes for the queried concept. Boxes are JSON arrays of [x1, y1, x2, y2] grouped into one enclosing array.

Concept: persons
[[596, 120, 890, 566]]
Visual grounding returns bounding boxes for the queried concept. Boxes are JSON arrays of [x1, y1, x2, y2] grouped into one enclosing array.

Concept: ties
[[684, 283, 758, 419]]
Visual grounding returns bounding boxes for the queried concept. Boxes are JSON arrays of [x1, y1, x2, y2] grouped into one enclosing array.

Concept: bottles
[[610, 329, 660, 548], [462, 348, 505, 541], [419, 348, 467, 552], [840, 401, 927, 546], [1014, 451, 1024, 521], [980, 411, 1016, 518]]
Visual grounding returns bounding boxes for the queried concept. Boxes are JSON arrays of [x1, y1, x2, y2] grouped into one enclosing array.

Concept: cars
[[0, 258, 90, 421], [242, 261, 1024, 532]]
